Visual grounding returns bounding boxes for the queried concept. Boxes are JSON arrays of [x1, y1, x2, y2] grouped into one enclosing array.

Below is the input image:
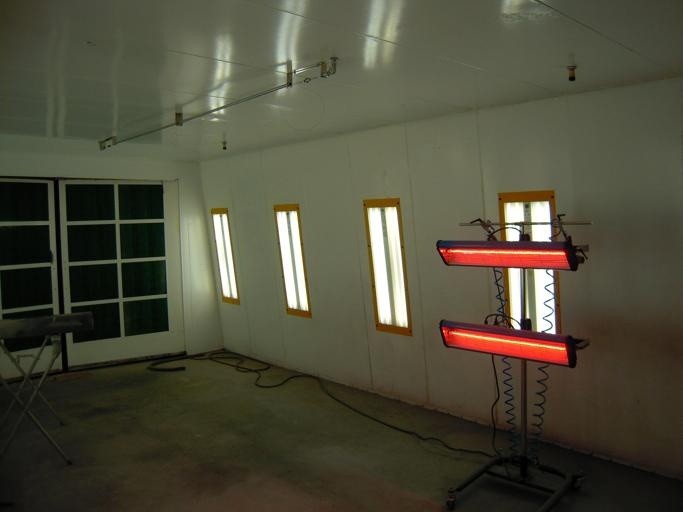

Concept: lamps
[[437, 237, 579, 272], [439, 318, 577, 368]]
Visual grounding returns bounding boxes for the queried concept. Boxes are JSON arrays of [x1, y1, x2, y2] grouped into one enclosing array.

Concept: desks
[[0, 311, 94, 465]]
